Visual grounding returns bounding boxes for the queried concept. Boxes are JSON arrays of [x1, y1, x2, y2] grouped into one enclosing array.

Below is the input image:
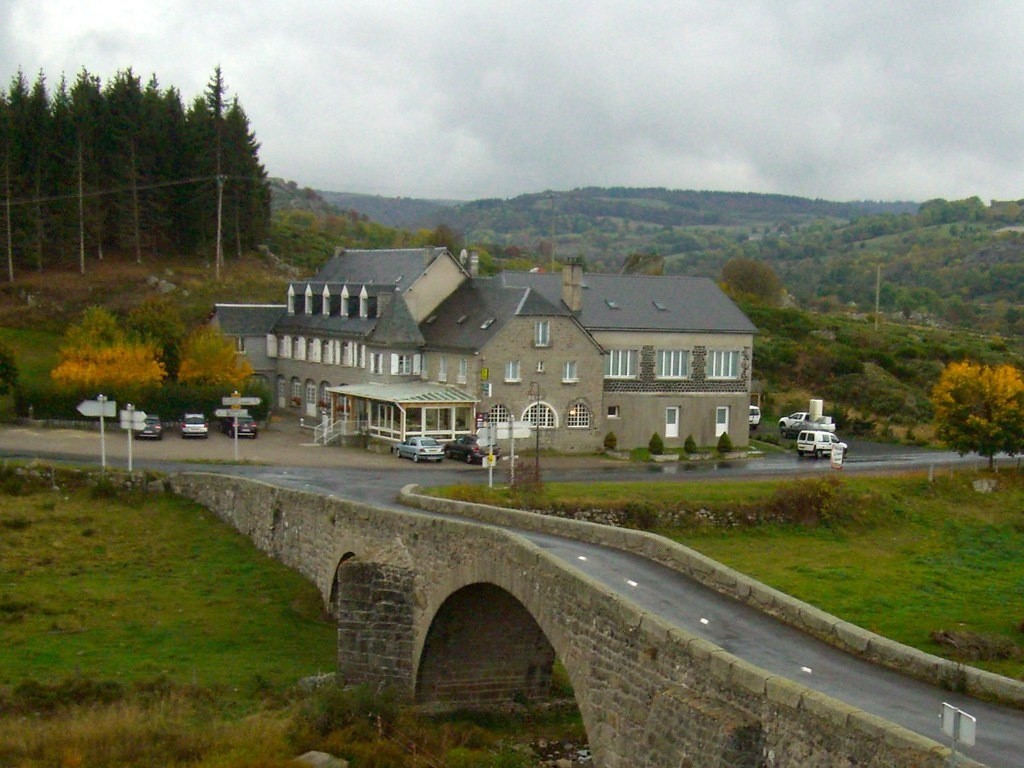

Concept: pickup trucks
[[779, 413, 837, 433]]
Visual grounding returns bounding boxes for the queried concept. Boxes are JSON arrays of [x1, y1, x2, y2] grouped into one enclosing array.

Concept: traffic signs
[[221, 396, 264, 407], [212, 408, 249, 418]]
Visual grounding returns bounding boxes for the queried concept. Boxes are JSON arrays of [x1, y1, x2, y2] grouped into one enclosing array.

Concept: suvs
[[748, 406, 761, 431], [179, 412, 209, 439], [134, 414, 163, 441]]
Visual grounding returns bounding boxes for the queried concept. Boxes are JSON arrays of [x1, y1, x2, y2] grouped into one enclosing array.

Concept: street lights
[[527, 380, 540, 475]]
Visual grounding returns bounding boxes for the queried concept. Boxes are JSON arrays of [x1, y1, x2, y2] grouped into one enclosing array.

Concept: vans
[[796, 429, 848, 460]]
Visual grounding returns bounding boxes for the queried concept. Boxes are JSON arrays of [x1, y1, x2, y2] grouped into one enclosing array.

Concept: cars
[[220, 415, 257, 440], [780, 421, 827, 440], [444, 433, 502, 465], [395, 436, 444, 464]]
